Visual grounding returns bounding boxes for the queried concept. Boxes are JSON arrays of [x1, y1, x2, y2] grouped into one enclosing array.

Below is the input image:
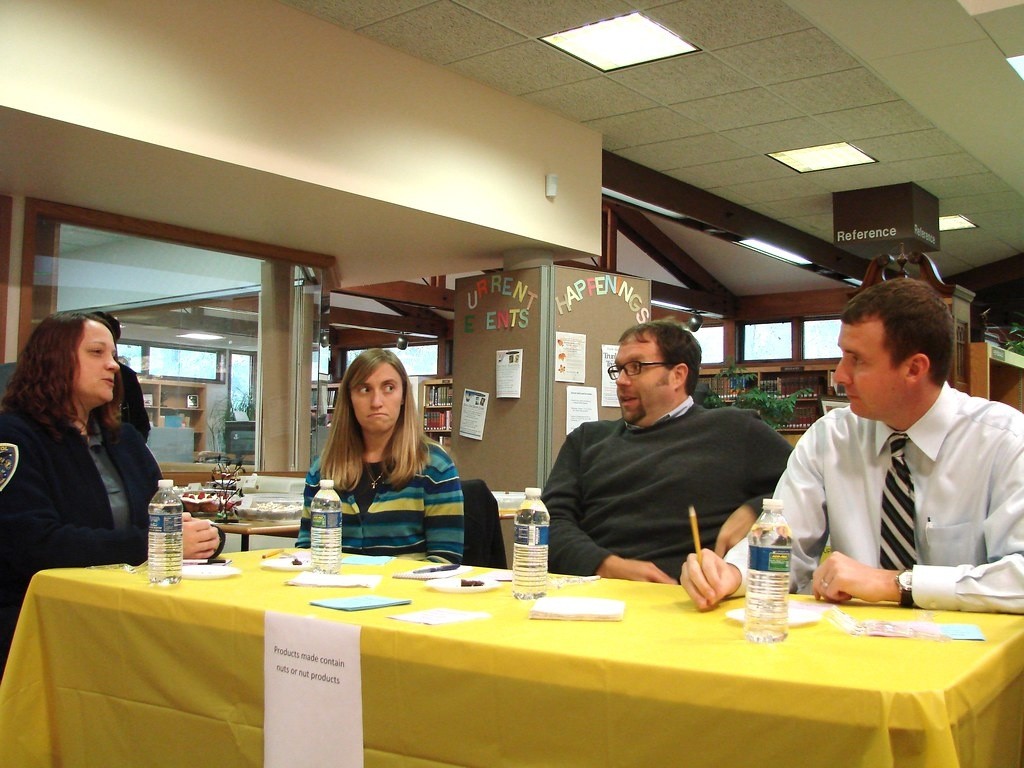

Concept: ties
[[877, 433, 917, 570]]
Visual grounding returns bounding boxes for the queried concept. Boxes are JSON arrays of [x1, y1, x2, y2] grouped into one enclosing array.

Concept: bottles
[[147, 479, 184, 586], [745, 499, 792, 645], [513, 488, 549, 602], [310, 479, 343, 575]]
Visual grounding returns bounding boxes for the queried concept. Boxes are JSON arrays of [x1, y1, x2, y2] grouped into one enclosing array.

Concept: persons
[[295, 349, 464, 565], [0, 310, 227, 682], [681, 277, 1024, 611], [540, 319, 795, 584], [88, 311, 151, 443]]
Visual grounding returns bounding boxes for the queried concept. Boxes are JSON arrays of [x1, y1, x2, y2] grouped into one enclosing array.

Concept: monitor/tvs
[[225, 421, 256, 460]]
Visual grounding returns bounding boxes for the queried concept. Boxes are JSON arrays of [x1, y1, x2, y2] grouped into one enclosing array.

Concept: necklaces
[[365, 462, 386, 489]]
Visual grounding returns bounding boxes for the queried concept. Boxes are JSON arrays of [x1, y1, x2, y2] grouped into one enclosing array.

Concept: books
[[696, 374, 826, 428], [159, 414, 190, 427], [424, 385, 453, 445], [144, 393, 153, 406], [830, 371, 847, 396], [311, 390, 336, 424]]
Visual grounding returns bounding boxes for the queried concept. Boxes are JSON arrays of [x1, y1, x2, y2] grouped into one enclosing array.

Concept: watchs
[[895, 568, 914, 608]]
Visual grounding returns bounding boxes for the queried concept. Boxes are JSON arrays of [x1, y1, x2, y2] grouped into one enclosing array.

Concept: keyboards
[[230, 460, 255, 465]]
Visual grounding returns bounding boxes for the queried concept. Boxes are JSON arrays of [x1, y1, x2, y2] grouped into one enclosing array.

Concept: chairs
[[460, 480, 508, 569]]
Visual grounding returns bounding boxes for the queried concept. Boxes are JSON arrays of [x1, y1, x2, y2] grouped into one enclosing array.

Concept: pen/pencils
[[411, 562, 461, 573], [183, 559, 226, 565], [687, 504, 704, 564], [263, 548, 285, 558]]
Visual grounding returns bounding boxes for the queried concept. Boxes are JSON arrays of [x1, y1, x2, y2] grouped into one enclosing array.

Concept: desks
[[196, 513, 519, 552], [0, 552, 1024, 768]]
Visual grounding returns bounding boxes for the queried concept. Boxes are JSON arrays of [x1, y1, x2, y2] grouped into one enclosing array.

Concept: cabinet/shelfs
[[420, 379, 453, 446], [135, 380, 209, 460], [694, 365, 844, 431]]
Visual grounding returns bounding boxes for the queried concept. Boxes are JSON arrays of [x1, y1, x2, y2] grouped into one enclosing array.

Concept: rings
[[822, 579, 828, 587]]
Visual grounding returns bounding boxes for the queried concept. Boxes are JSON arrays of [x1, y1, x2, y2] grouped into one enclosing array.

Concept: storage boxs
[[240, 493, 305, 524], [491, 491, 527, 514]]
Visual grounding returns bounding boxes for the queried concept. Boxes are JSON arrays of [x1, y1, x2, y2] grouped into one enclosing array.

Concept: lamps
[[688, 309, 704, 333], [397, 331, 410, 350]]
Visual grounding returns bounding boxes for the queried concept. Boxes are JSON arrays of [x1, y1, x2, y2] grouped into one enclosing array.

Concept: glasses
[[607, 360, 679, 380]]
